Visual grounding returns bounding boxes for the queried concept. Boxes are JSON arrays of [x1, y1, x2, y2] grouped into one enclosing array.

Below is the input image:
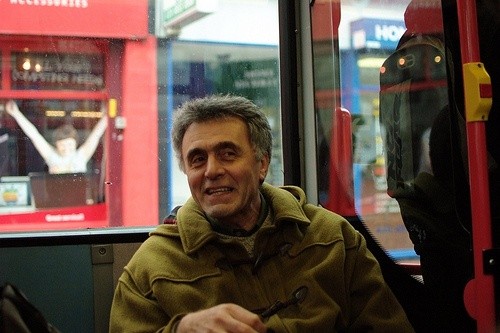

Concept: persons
[[5, 99, 107, 174], [108, 94, 416, 333], [376, 0, 474, 333]]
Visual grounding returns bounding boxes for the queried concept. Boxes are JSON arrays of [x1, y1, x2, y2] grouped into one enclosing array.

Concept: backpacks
[[379, 35, 470, 198]]
